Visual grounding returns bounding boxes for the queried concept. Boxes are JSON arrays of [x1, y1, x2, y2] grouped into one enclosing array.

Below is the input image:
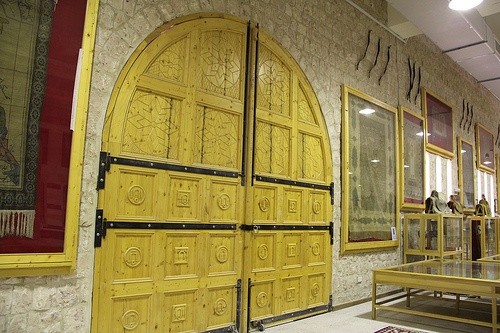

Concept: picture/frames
[[340, 83, 402, 256], [420, 84, 457, 159], [457, 136, 476, 214], [0, 0, 101, 278], [397, 106, 426, 212], [476, 121, 496, 175]]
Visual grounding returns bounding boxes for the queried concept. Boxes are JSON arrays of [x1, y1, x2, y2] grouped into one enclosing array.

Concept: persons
[[466, 204, 491, 280], [426, 190, 443, 239], [479, 199, 493, 243], [448, 192, 464, 216]]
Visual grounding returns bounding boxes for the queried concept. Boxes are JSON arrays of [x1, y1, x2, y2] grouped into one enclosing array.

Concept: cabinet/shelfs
[[468, 216, 500, 262], [404, 213, 464, 298]]
